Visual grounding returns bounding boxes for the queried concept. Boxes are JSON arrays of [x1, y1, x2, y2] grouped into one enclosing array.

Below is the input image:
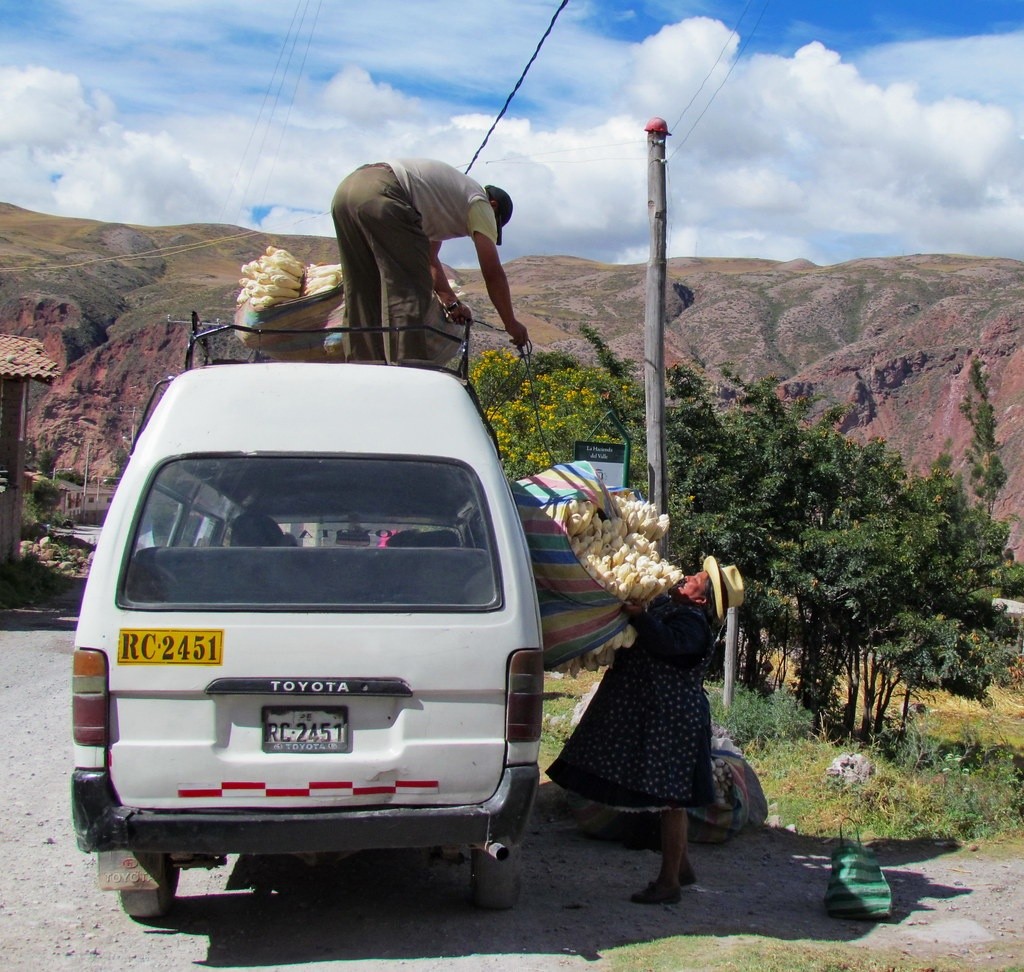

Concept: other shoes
[[631, 886, 681, 904], [649, 864, 696, 888]]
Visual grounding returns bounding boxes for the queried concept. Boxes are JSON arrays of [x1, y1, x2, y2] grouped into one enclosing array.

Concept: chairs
[[384, 528, 461, 548], [228, 513, 298, 547]]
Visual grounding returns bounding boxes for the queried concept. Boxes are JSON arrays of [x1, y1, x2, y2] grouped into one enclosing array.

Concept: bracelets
[[444, 299, 460, 311]]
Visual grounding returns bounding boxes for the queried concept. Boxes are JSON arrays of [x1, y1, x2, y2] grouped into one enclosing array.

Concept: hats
[[703, 555, 744, 625], [484, 185, 513, 245]]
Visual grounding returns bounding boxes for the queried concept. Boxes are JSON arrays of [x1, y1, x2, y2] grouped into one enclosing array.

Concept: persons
[[330, 157, 528, 363], [544, 555, 744, 904]]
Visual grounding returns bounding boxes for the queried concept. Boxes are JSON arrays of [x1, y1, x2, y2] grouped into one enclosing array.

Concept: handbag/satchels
[[824, 817, 892, 919]]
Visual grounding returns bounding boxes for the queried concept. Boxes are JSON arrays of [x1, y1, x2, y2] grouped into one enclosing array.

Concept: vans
[[70, 316, 545, 923]]
